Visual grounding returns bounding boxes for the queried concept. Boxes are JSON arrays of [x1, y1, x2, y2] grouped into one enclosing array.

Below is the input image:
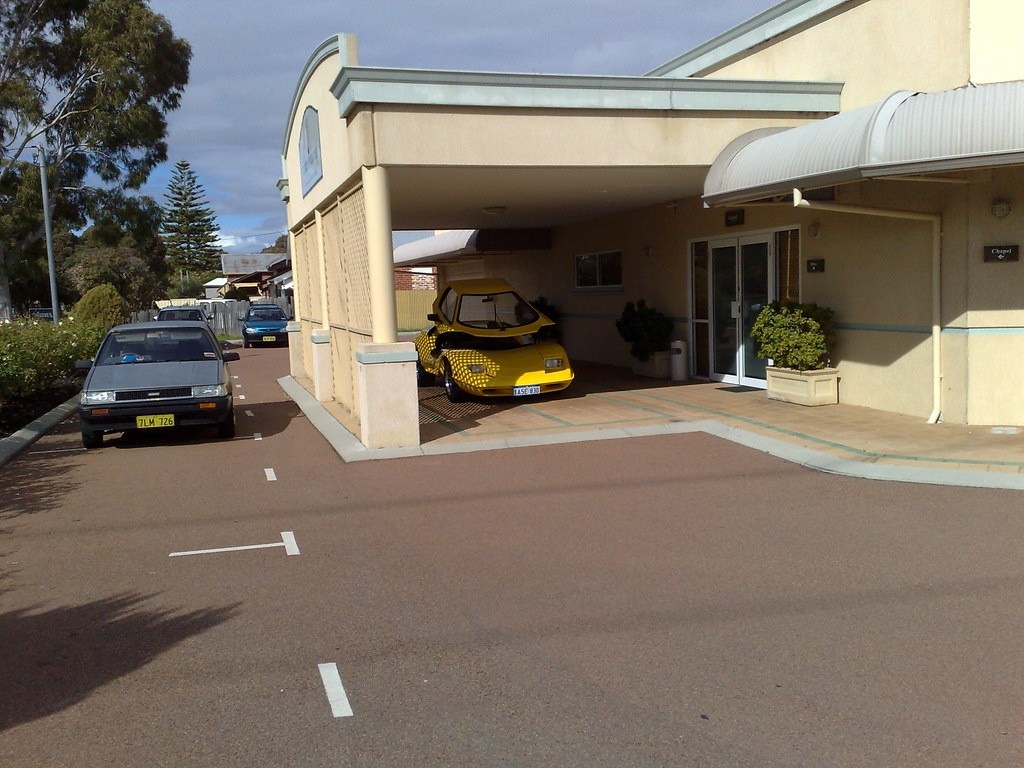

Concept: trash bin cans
[[670, 339, 688, 383]]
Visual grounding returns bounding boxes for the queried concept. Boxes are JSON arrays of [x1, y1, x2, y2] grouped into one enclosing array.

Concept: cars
[[154, 306, 215, 321], [76, 321, 234, 448], [242, 305, 295, 348]]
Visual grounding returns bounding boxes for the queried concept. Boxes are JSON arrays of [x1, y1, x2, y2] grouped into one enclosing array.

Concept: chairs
[[189, 312, 197, 320], [166, 313, 175, 320], [176, 340, 204, 361]]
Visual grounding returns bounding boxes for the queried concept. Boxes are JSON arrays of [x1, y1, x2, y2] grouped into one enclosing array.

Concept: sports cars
[[414, 277, 578, 404]]
